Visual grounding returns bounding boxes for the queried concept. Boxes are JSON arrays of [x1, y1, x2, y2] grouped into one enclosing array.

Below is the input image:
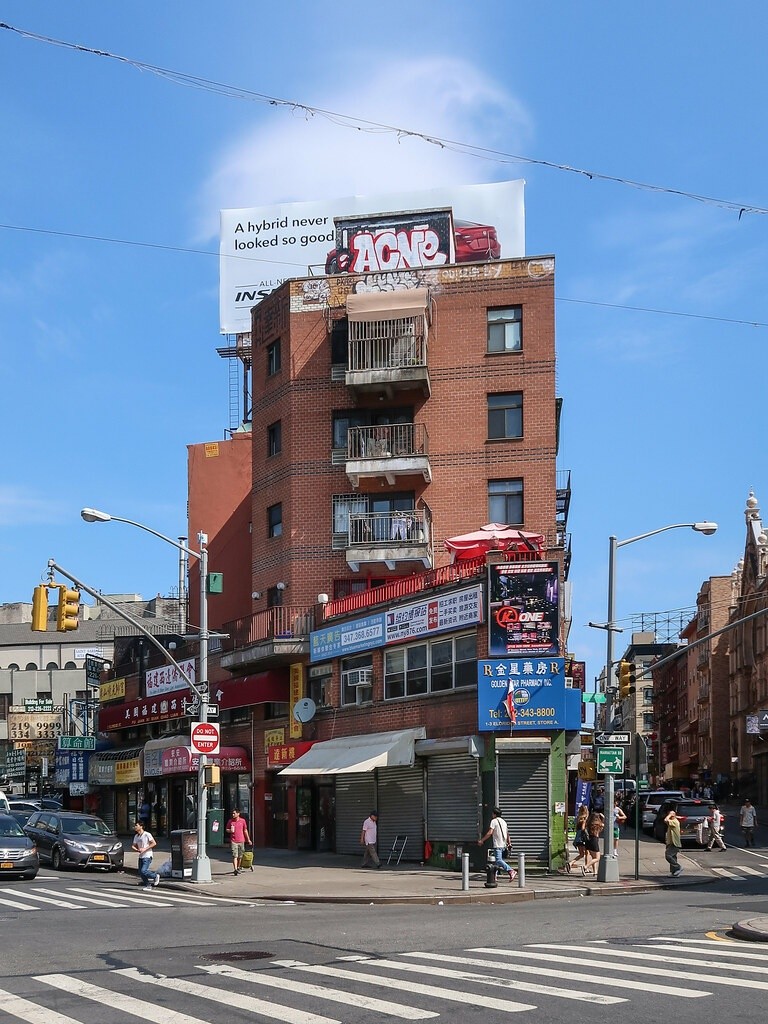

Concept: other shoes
[[509, 870, 517, 882], [719, 848, 726, 852], [593, 873, 598, 877], [377, 864, 382, 868], [154, 874, 160, 886], [673, 867, 683, 876], [704, 848, 711, 852], [238, 868, 241, 874], [142, 885, 152, 891], [360, 864, 372, 869], [667, 872, 680, 878], [581, 866, 587, 877], [566, 863, 572, 874], [234, 870, 238, 875]]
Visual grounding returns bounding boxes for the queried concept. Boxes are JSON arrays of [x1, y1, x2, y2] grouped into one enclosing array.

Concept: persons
[[478, 807, 517, 882], [226, 808, 252, 876], [662, 810, 683, 875], [138, 799, 152, 832], [700, 805, 727, 852], [131, 820, 160, 888], [738, 797, 758, 847], [592, 786, 624, 812], [686, 780, 740, 805], [360, 811, 383, 869], [572, 804, 605, 877], [614, 800, 627, 857], [679, 784, 690, 792]]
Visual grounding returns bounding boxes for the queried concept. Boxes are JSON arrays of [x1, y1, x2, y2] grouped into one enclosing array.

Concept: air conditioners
[[158, 721, 170, 734], [170, 720, 182, 734], [180, 717, 196, 729], [347, 669, 372, 686]]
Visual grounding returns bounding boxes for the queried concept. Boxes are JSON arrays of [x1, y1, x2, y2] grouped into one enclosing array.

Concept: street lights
[[598, 521, 720, 884], [80, 505, 212, 883]]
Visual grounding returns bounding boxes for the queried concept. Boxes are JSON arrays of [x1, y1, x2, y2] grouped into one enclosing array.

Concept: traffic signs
[[184, 703, 220, 718], [597, 746, 625, 775], [593, 729, 632, 746]]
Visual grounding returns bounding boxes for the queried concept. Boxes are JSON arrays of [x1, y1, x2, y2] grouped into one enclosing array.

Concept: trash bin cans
[[206, 808, 225, 847], [171, 829, 197, 879]]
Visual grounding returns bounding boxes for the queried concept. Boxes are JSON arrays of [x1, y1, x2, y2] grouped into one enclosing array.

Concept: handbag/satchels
[[502, 849, 511, 859]]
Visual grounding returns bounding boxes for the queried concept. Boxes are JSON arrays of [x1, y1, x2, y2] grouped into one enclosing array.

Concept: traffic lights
[[619, 661, 636, 701], [56, 586, 81, 632]]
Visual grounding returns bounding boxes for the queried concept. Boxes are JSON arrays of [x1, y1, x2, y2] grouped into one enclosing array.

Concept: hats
[[744, 798, 750, 803]]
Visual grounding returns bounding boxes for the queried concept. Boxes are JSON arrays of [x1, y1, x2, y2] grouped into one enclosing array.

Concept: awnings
[[143, 732, 191, 777], [278, 726, 427, 775], [96, 669, 290, 733], [87, 745, 145, 785], [161, 745, 252, 775]]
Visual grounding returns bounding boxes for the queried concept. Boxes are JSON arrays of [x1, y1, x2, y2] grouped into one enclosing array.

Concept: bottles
[[231, 825, 234, 832]]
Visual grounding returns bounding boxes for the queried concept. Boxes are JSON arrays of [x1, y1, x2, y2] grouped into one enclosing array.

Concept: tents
[[442, 521, 546, 565]]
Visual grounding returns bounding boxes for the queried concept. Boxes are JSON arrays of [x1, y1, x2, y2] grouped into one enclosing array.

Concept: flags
[[502, 678, 516, 724]]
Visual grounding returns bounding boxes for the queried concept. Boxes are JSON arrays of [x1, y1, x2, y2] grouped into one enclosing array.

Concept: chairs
[[386, 835, 408, 865]]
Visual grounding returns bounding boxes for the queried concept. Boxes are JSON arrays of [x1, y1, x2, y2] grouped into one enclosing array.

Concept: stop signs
[[190, 721, 220, 754]]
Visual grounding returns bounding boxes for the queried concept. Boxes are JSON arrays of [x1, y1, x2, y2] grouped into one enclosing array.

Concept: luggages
[[241, 845, 254, 872]]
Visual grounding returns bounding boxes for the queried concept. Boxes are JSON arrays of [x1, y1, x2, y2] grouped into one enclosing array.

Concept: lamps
[[251, 591, 263, 600], [277, 582, 289, 591]]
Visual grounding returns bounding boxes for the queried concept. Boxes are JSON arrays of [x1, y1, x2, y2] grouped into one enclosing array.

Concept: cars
[[651, 797, 726, 847], [592, 778, 684, 834], [0, 809, 41, 881], [22, 808, 124, 873], [0, 790, 64, 830]]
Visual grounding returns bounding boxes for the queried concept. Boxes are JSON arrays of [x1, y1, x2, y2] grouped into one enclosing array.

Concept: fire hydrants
[[484, 863, 500, 888]]
